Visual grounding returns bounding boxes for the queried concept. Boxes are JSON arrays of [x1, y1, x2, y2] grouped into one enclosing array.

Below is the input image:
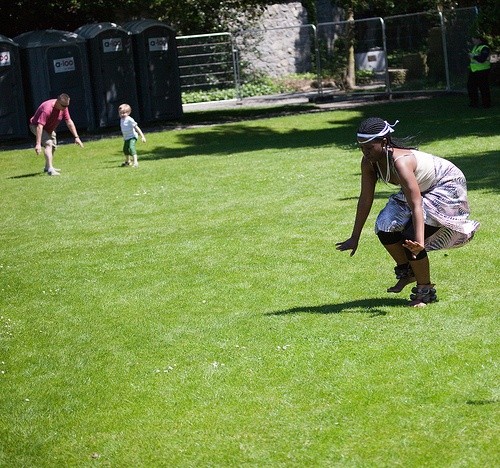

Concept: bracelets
[[75, 136, 79, 138]]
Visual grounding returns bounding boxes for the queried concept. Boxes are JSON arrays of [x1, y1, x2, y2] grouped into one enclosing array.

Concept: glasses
[[60, 104, 70, 107]]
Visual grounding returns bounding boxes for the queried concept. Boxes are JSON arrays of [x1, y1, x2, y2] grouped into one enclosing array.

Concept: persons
[[30, 94, 83, 176], [468, 34, 491, 109], [118, 104, 147, 167], [335, 118, 480, 307]]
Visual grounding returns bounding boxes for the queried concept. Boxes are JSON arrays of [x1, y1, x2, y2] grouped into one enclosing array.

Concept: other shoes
[[44, 167, 61, 172], [122, 161, 131, 166], [47, 171, 59, 175]]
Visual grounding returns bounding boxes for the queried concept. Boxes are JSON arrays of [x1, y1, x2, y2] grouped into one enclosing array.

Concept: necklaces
[[376, 149, 390, 181]]
